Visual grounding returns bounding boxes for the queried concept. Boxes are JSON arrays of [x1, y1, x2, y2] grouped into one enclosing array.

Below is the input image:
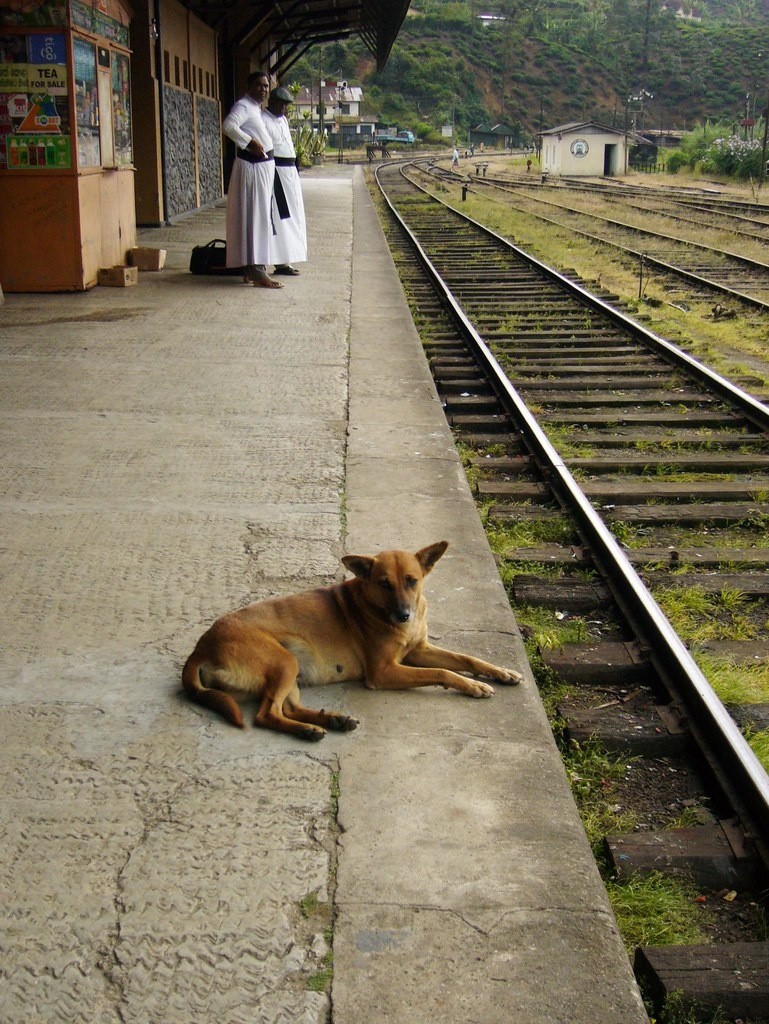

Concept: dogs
[[182, 541, 520, 740]]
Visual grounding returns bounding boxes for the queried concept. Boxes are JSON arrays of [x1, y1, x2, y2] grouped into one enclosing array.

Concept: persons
[[451, 149, 460, 173], [221, 71, 307, 288], [469, 142, 474, 156]]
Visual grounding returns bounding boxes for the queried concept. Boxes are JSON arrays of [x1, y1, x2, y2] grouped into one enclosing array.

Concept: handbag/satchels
[[190, 239, 246, 276], [454, 160, 458, 166]]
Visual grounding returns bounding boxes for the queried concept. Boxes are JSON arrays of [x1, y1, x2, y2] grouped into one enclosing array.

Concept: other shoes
[[253, 276, 285, 288], [244, 275, 255, 283], [273, 265, 300, 275]]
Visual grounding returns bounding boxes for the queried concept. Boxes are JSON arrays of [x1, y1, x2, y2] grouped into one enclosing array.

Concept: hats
[[270, 88, 294, 103]]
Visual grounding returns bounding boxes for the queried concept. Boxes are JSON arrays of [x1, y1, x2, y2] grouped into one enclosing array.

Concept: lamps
[[149, 18, 158, 39]]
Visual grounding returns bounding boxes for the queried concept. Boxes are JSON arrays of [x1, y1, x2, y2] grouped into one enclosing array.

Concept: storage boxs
[[131, 246, 166, 270], [97, 266, 137, 286]]
